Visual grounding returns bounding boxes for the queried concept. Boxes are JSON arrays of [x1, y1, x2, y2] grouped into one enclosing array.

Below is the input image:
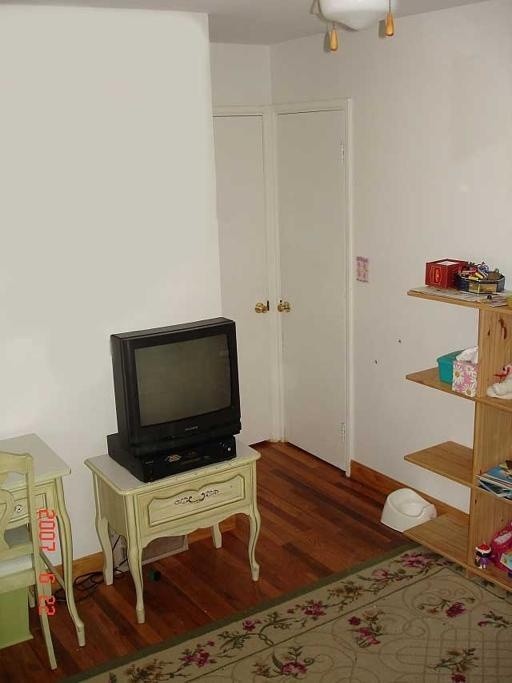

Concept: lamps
[[311, 0, 399, 53]]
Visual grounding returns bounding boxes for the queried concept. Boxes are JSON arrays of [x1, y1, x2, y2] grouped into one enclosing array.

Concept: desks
[[0, 433, 85, 649], [84, 438, 262, 624]]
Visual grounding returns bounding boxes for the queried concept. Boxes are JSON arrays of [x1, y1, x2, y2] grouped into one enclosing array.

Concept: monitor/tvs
[[110, 317, 242, 457]]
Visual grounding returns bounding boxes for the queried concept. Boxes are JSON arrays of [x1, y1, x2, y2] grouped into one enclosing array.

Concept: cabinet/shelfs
[[401, 285, 512, 594]]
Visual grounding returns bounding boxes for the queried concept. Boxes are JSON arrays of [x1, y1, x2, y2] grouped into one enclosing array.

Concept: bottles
[[475, 544, 491, 569]]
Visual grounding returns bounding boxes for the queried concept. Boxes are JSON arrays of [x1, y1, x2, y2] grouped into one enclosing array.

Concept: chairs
[[0, 450, 59, 671]]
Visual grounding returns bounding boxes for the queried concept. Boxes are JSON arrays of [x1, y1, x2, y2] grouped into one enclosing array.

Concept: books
[[475, 460, 511, 498]]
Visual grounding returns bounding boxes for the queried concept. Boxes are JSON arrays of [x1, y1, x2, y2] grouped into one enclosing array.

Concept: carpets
[[58, 541, 512, 682]]
